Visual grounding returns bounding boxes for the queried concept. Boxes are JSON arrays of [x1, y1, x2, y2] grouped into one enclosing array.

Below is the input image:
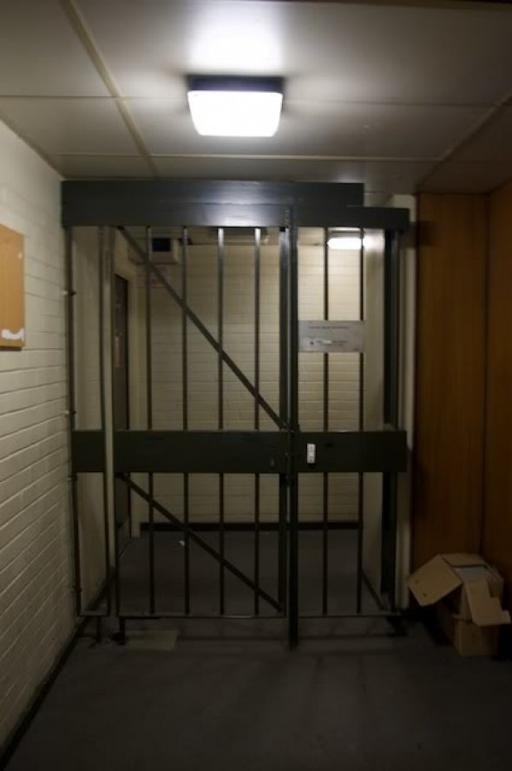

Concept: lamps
[[185, 74, 287, 139]]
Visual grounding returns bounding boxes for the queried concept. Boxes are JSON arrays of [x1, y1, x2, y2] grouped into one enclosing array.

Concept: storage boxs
[[405, 551, 510, 659]]
